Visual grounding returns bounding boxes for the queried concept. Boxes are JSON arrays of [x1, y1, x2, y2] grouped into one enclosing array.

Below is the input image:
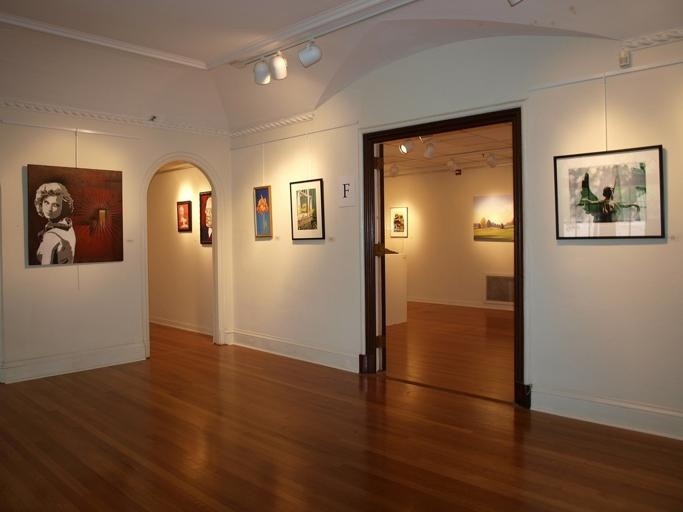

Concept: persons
[[33, 182, 76, 265], [201, 197, 212, 242], [588, 187, 621, 215]]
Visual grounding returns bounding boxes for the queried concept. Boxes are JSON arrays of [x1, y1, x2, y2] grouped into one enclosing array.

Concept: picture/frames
[[552, 145, 666, 239], [177, 201, 192, 232], [252, 178, 324, 241], [199, 191, 213, 245], [388, 206, 408, 239]]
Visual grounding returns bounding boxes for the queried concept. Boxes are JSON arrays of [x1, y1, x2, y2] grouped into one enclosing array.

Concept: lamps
[[423, 143, 435, 159], [399, 141, 414, 154], [252, 37, 321, 85]]
